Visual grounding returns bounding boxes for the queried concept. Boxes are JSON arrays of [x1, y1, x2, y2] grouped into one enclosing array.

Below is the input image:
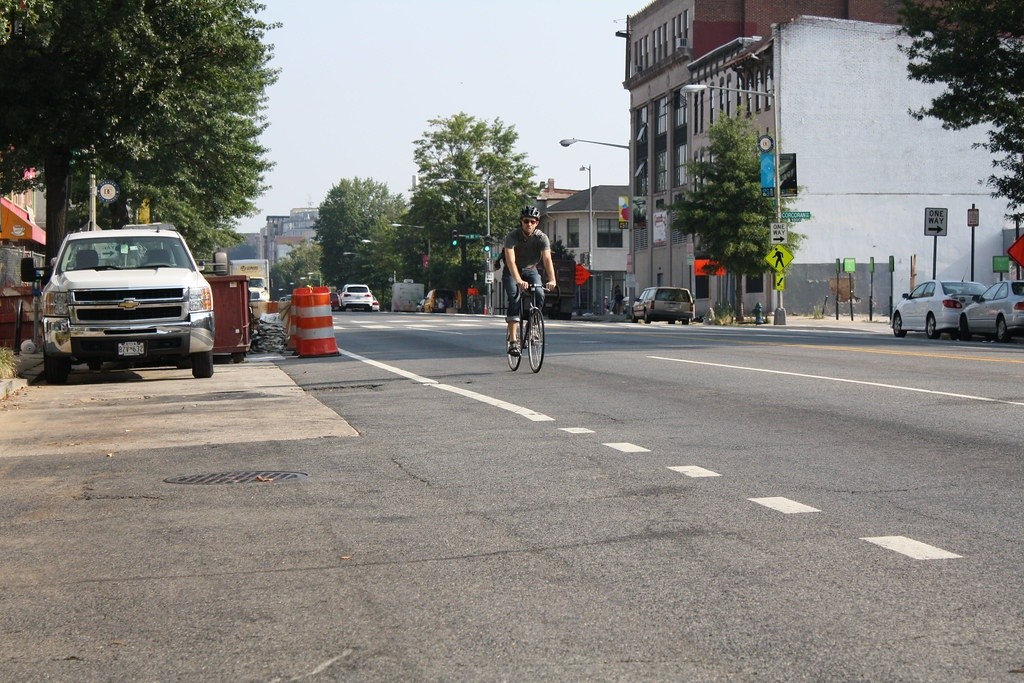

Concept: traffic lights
[[452, 229, 458, 246], [484, 236, 490, 252]]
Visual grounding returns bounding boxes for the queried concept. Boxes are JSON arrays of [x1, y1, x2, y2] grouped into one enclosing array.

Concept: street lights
[[360, 238, 396, 283], [392, 223, 431, 290], [436, 179, 492, 315], [680, 83, 787, 325], [559, 137, 635, 321], [307, 272, 322, 287], [578, 163, 593, 271]]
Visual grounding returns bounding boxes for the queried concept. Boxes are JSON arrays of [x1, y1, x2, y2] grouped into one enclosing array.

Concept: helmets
[[520, 205, 541, 220]]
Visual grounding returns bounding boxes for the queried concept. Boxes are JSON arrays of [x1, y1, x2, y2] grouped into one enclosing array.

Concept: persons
[[614, 285, 622, 315], [501, 205, 556, 356]]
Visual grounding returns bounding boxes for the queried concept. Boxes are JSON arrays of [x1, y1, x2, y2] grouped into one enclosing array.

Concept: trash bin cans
[[204, 275, 252, 364]]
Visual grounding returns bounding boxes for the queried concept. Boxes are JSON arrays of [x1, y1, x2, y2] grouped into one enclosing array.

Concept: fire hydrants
[[751, 301, 765, 325]]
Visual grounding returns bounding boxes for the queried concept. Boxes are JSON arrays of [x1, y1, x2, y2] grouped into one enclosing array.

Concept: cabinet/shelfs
[[206, 275, 252, 362]]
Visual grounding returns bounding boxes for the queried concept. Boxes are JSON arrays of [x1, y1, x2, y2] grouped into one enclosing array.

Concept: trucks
[[228, 259, 271, 301], [391, 279, 424, 312]]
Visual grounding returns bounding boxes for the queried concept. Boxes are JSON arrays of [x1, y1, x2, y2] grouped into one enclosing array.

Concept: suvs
[[340, 284, 372, 312], [632, 286, 695, 325], [19, 228, 228, 384]]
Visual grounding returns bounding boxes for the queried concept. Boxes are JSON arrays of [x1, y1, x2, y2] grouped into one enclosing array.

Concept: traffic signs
[[924, 207, 948, 237], [771, 223, 788, 245]]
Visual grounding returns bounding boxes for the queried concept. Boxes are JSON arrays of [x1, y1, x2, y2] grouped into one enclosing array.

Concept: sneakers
[[509, 344, 520, 356]]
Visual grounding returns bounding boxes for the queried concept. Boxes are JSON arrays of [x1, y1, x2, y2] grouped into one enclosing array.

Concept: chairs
[[73, 250, 99, 270], [149, 250, 170, 264]]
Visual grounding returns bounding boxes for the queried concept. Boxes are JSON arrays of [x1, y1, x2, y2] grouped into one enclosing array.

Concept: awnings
[[0, 199, 46, 245]]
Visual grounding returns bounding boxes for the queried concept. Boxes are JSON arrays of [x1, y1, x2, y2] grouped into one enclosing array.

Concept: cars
[[278, 294, 291, 302], [372, 295, 380, 312], [890, 279, 989, 340], [958, 279, 1024, 344], [330, 292, 342, 312], [416, 298, 427, 312], [424, 289, 456, 313]]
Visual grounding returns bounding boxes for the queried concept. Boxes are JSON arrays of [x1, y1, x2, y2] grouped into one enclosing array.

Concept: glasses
[[522, 219, 537, 224]]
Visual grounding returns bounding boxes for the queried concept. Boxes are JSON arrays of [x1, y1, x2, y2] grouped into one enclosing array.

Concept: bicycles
[[507, 283, 553, 373]]
